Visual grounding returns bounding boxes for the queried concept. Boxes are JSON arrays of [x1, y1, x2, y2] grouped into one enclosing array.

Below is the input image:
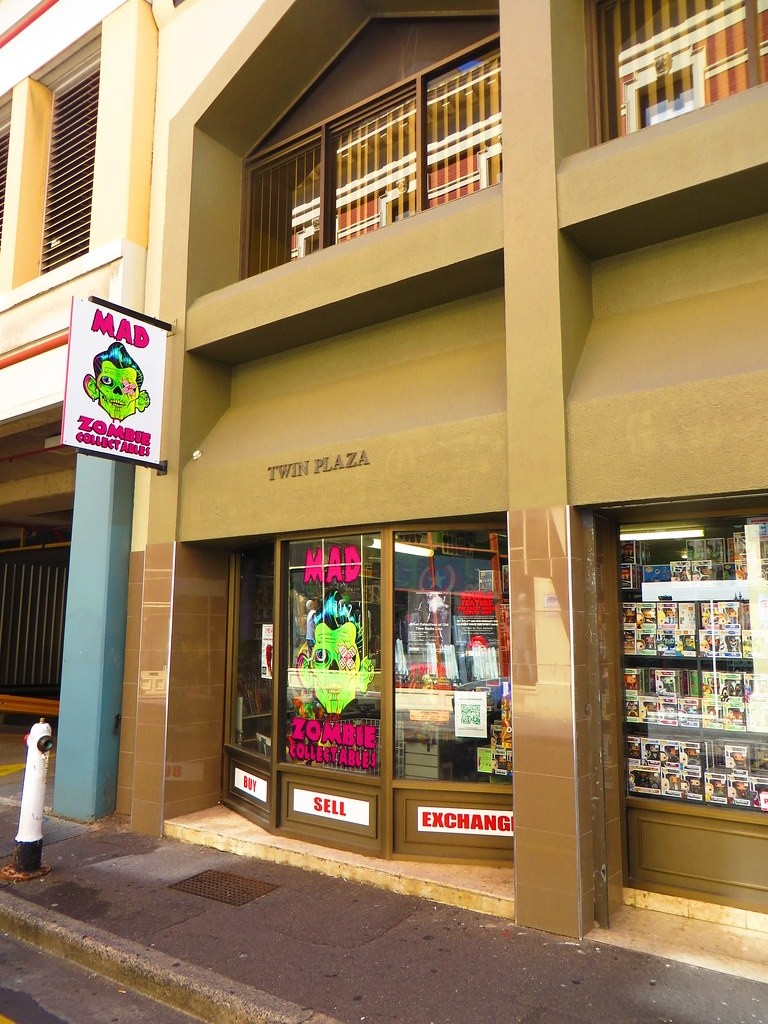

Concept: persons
[[306, 597, 320, 653]]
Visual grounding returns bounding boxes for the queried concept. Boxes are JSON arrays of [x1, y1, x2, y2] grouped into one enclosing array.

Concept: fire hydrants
[[0, 715, 54, 882]]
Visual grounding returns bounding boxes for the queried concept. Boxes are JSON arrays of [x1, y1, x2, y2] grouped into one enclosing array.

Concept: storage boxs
[[501, 565, 510, 593], [344, 591, 356, 601], [478, 570, 501, 592], [619, 515, 768, 813], [365, 585, 380, 603]]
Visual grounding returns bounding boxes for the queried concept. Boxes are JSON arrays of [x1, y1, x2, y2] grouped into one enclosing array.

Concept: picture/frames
[[255, 574, 274, 624]]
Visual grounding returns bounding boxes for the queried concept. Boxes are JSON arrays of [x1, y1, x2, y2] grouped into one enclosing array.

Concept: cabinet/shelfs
[[622, 598, 768, 812]]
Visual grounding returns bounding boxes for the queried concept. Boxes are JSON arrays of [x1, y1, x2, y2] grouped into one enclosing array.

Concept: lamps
[[367, 538, 434, 557], [620, 526, 705, 542]]
